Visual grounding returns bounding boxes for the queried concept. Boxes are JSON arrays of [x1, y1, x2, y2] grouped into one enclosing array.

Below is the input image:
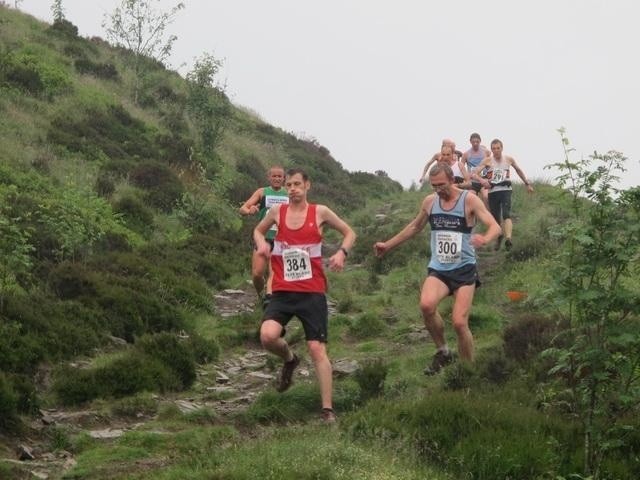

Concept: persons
[[253, 167, 356, 425], [418, 139, 463, 182], [471, 139, 533, 251], [374, 161, 502, 375], [440, 146, 473, 188], [240, 166, 291, 307], [459, 133, 491, 207]]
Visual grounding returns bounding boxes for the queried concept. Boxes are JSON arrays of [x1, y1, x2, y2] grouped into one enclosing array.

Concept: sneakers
[[322, 407, 336, 423], [423, 349, 453, 376], [262, 293, 272, 309], [505, 239, 513, 251], [275, 355, 299, 393], [494, 234, 504, 251]]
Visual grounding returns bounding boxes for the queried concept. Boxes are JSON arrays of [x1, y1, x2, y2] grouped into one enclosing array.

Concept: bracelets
[[339, 246, 350, 255], [525, 178, 531, 185]]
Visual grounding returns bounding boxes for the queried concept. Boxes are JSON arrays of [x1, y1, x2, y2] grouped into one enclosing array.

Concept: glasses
[[429, 182, 449, 190]]
